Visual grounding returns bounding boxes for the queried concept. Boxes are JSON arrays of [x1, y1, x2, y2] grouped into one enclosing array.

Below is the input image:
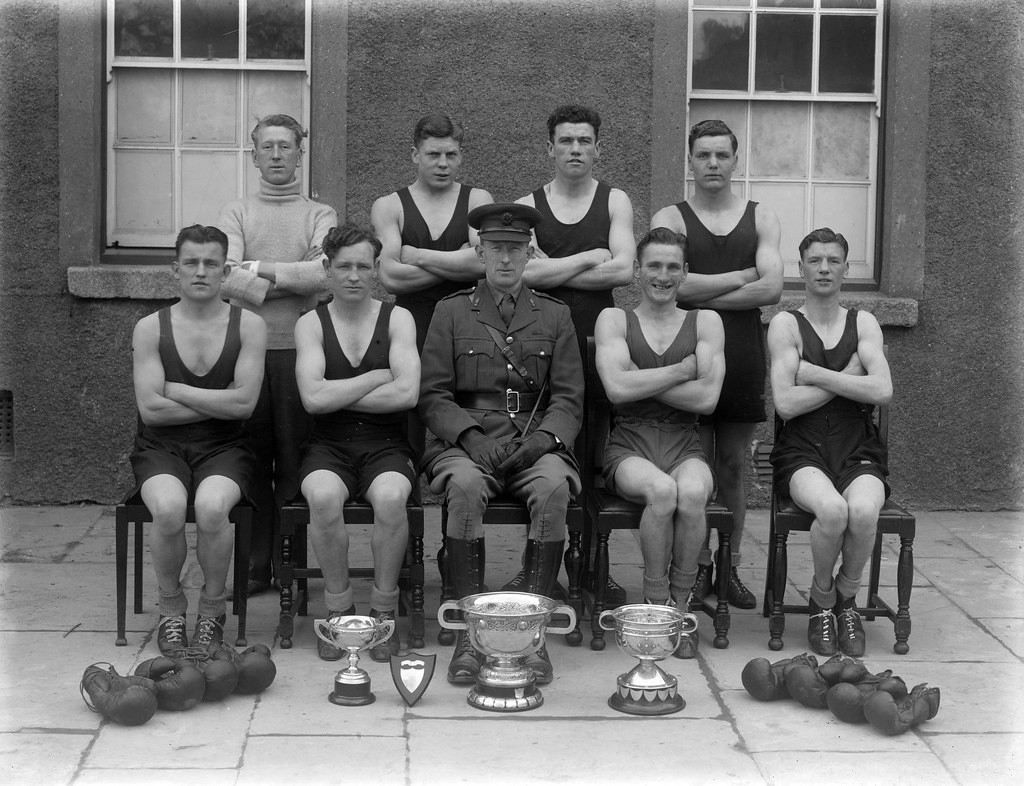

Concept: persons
[[593, 226, 727, 658], [650, 119, 784, 611], [499, 104, 635, 605], [372, 110, 495, 595], [130, 224, 267, 658], [218, 115, 337, 601], [418, 204, 585, 685], [767, 228, 894, 658], [292, 222, 421, 663]]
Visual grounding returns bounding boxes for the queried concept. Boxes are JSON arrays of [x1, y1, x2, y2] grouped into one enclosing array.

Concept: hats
[[467, 203, 542, 241]]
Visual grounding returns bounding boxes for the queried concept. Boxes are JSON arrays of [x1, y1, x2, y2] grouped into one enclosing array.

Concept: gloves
[[741, 652, 940, 734], [80, 642, 277, 728], [458, 429, 508, 473], [498, 431, 556, 474]]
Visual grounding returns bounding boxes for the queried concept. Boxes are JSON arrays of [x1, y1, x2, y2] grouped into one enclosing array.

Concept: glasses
[[640, 266, 685, 277]]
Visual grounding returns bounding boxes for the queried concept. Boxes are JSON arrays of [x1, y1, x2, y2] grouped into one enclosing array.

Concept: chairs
[[113, 337, 735, 649], [764, 344, 917, 654]]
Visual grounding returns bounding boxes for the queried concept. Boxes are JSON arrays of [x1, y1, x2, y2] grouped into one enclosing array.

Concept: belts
[[454, 392, 552, 412]]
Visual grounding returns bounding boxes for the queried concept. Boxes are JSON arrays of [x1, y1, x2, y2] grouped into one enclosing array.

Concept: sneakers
[[502, 568, 527, 592], [190, 612, 227, 659], [156, 613, 188, 659], [585, 569, 626, 604], [808, 596, 844, 657], [319, 603, 356, 661], [368, 608, 400, 662], [714, 564, 757, 610], [686, 562, 714, 613], [834, 590, 865, 659], [643, 593, 699, 658]]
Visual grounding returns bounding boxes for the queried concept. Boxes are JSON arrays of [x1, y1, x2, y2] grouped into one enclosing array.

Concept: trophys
[[436, 590, 576, 713], [599, 603, 698, 715], [314, 615, 395, 707]]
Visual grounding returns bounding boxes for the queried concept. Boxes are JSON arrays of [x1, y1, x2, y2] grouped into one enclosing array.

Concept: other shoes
[[228, 577, 267, 601]]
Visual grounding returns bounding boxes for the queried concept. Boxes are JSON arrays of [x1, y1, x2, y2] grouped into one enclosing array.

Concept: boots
[[518, 539, 565, 684], [442, 536, 486, 684]]
